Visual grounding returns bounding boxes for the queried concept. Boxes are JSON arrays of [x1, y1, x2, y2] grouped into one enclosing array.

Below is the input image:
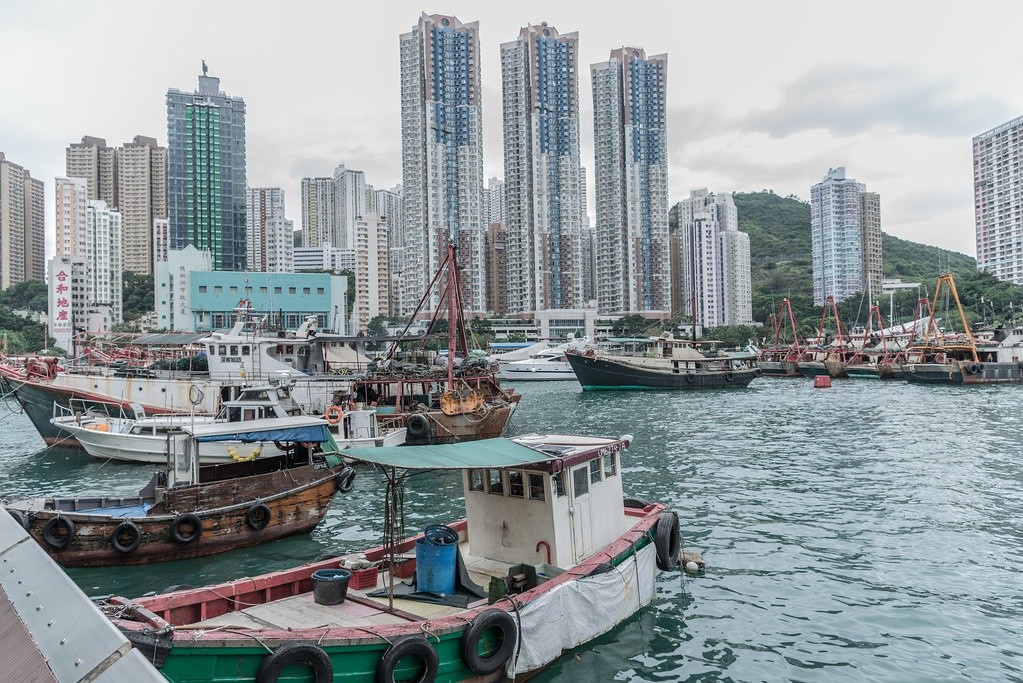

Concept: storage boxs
[[389, 553, 417, 578], [338, 560, 379, 590], [310, 567, 352, 605]]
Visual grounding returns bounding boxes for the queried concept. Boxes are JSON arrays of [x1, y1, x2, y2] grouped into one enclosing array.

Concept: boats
[[10, 412, 352, 569], [52, 383, 411, 468], [0, 268, 452, 453], [90, 429, 682, 681]]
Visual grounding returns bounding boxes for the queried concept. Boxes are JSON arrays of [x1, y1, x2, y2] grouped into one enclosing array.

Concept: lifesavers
[[300, 441, 318, 448], [325, 406, 343, 424], [274, 440, 299, 451]]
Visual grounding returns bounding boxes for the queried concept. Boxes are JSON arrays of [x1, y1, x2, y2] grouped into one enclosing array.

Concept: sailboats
[[489, 272, 1022, 388], [358, 242, 520, 444]]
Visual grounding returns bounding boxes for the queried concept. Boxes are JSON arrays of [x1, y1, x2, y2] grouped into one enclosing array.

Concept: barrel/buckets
[[311, 568, 351, 606], [415, 538, 457, 596]]
